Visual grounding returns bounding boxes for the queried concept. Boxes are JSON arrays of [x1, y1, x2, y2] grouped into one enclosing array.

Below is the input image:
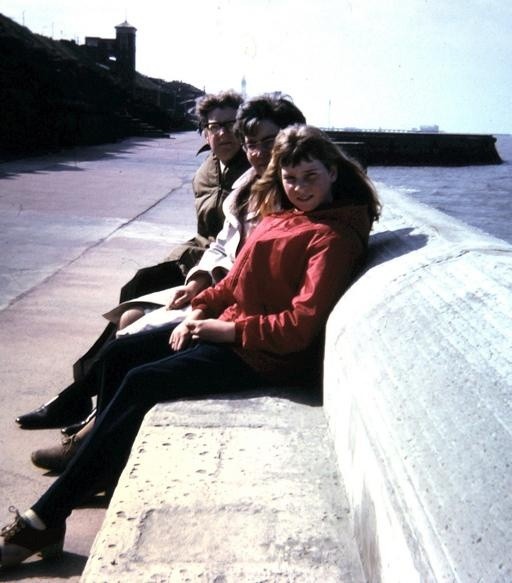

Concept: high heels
[[0, 517, 65, 569]]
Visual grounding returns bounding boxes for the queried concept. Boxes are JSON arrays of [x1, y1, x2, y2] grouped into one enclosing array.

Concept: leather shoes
[[16, 394, 95, 470]]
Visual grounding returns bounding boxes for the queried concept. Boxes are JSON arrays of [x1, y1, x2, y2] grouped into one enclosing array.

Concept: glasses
[[206, 121, 235, 133], [241, 140, 274, 153]]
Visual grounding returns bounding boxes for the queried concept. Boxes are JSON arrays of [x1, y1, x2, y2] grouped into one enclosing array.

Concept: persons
[[0, 124, 380, 571], [15, 93, 244, 428], [31, 96, 306, 471]]
